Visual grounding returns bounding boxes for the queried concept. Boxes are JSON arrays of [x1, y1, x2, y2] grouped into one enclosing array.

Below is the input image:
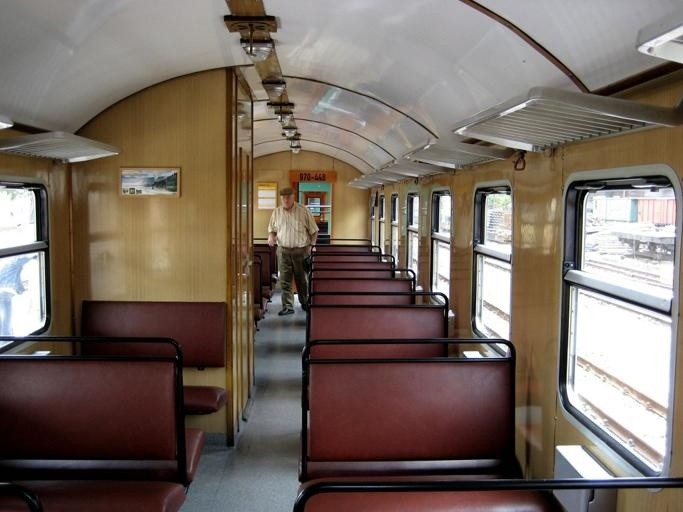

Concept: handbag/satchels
[[302, 256, 312, 274]]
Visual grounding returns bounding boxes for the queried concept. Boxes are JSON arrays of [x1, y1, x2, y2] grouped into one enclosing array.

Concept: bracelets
[[309, 244, 315, 246]]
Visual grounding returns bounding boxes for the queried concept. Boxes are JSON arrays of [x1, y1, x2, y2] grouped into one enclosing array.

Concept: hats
[[280, 187, 294, 195]]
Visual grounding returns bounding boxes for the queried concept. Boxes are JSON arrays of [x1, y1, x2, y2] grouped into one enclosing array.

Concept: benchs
[[0, 298, 237, 512], [291, 236, 566, 512], [252, 237, 278, 332]]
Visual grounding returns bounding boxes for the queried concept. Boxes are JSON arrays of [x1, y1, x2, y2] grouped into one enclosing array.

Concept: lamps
[[225, 13, 279, 64], [261, 78, 302, 155]]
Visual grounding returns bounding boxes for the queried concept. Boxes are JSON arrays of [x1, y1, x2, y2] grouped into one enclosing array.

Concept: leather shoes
[[279, 308, 294, 315], [302, 304, 306, 310]]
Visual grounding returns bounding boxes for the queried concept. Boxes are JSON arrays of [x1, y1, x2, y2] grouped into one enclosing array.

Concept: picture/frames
[[118, 166, 181, 198], [255, 181, 279, 210]]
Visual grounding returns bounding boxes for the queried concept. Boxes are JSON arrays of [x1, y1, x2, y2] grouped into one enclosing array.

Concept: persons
[[265, 187, 319, 315]]
